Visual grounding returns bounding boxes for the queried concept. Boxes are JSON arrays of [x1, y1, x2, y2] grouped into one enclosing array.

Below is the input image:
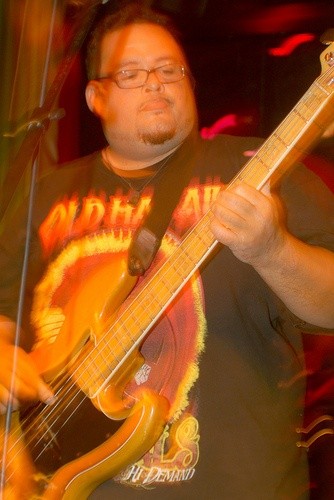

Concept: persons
[[0, 3, 334, 500]]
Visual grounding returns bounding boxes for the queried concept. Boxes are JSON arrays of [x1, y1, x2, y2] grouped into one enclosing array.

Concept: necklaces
[[100, 146, 182, 206]]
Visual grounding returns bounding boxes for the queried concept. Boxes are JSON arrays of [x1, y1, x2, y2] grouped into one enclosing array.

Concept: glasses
[[93, 63, 191, 89]]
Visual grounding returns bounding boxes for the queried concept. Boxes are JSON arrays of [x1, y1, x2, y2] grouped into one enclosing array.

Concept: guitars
[[1, 39, 333, 499]]
